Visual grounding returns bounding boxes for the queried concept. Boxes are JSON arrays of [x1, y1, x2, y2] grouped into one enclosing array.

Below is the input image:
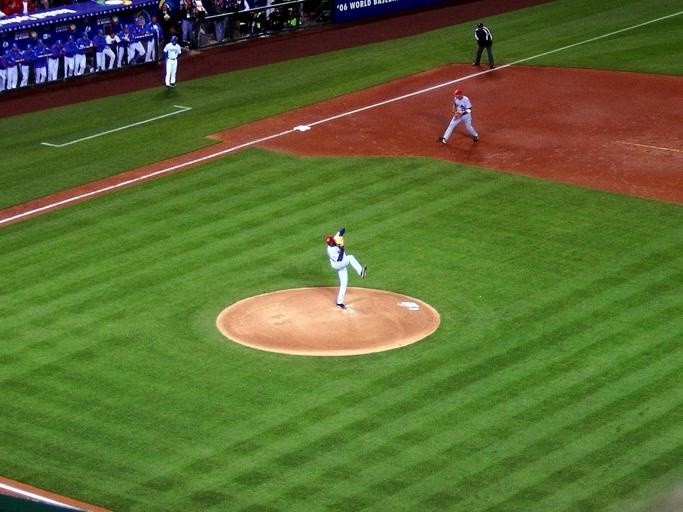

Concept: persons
[[0, 0, 326, 89], [437, 90, 479, 146], [471, 23, 494, 69], [325, 228, 366, 309]]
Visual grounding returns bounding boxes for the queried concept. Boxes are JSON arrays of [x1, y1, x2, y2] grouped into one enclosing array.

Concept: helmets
[[170, 35, 177, 42], [453, 89, 463, 96], [3, 17, 141, 58], [326, 236, 334, 246]]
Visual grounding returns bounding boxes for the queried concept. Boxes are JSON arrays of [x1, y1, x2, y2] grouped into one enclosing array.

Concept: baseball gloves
[[335, 237, 344, 247], [454, 112, 462, 119]]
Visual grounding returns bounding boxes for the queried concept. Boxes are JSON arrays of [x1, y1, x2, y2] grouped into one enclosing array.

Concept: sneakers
[[472, 136, 478, 141], [336, 304, 347, 310], [439, 136, 446, 144], [361, 264, 367, 279]]
